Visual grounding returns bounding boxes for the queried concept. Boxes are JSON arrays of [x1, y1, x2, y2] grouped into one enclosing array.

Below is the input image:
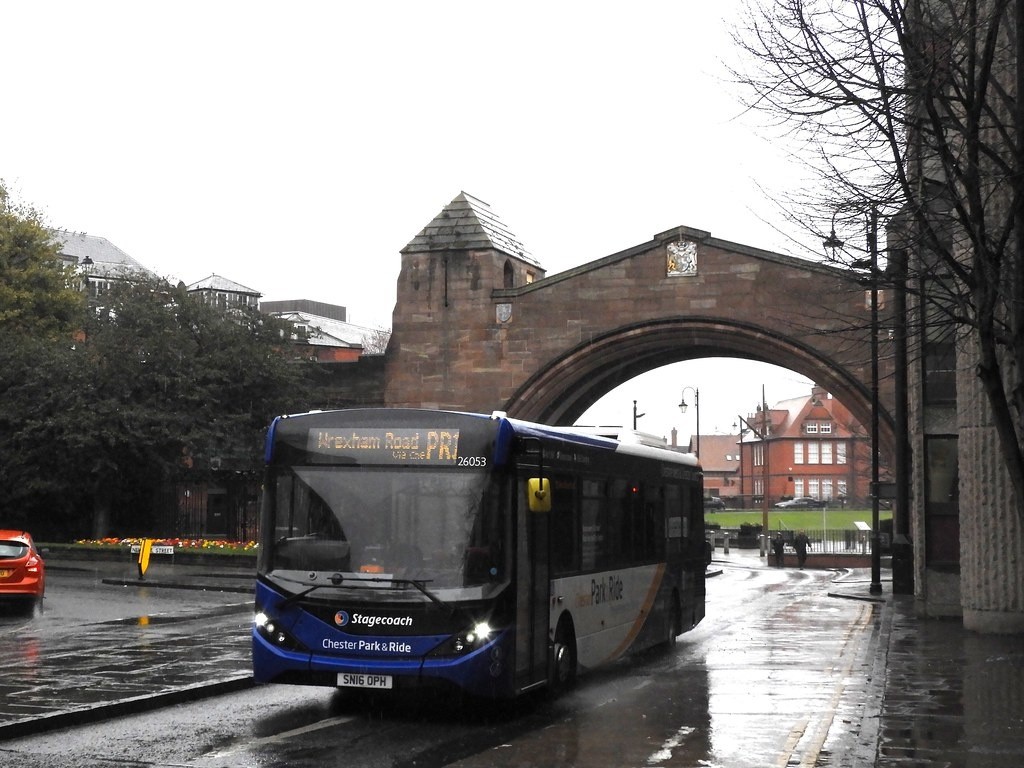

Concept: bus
[[252, 408, 706, 716]]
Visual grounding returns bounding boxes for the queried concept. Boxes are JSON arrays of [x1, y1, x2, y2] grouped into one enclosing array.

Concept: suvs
[[0, 529, 44, 617], [703, 497, 726, 512]]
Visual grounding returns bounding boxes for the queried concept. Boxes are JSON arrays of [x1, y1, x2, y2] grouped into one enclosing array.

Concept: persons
[[792, 529, 813, 570], [705, 537, 712, 571], [773, 532, 785, 568]]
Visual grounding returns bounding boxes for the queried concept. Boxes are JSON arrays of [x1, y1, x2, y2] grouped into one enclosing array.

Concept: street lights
[[679, 385, 699, 461]]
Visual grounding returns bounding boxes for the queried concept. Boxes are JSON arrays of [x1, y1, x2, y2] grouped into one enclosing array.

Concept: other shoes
[[800, 566, 804, 570]]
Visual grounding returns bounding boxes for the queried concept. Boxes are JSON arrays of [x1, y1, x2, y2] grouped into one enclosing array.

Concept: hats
[[777, 532, 781, 535], [800, 528, 805, 533]]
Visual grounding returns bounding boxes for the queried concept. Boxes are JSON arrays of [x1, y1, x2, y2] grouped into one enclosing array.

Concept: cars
[[774, 497, 827, 510]]
